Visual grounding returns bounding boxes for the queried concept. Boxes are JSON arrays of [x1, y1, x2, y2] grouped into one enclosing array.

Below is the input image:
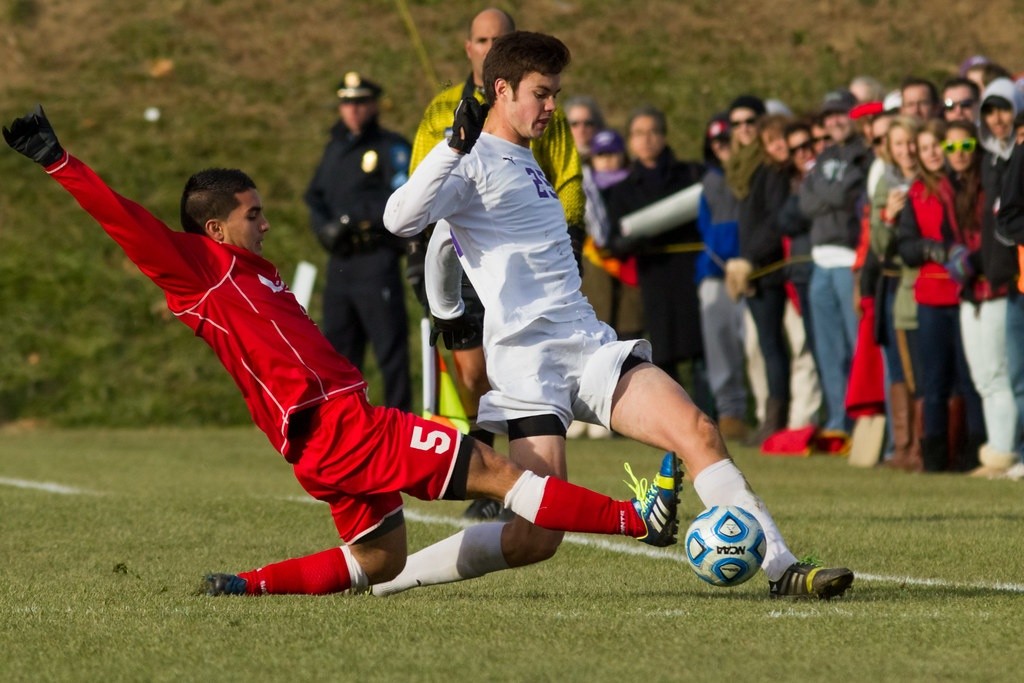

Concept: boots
[[883, 383, 965, 473]]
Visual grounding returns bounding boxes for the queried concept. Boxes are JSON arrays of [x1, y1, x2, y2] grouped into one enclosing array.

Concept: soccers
[[684, 504, 768, 587]]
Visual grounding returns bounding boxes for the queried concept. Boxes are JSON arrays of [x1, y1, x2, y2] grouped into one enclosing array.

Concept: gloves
[[448, 96, 490, 154], [2, 104, 64, 167]]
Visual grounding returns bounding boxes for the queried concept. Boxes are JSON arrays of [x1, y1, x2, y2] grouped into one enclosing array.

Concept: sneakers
[[464, 499, 501, 520], [207, 572, 247, 597], [769, 562, 854, 601], [622, 451, 684, 548]]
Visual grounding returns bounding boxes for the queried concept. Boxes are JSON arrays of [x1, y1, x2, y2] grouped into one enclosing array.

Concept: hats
[[727, 95, 766, 117], [960, 57, 988, 78], [591, 131, 624, 154], [819, 91, 857, 113], [335, 72, 383, 104]]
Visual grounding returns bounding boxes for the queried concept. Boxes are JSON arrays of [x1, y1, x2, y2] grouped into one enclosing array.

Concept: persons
[[407, 6, 585, 522], [565, 60, 1024, 480], [2, 103, 686, 595], [302, 71, 413, 413], [342, 31, 855, 599]]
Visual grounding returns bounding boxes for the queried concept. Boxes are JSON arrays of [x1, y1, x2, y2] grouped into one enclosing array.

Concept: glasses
[[568, 119, 593, 126], [731, 118, 755, 127], [872, 134, 888, 147], [941, 98, 974, 110], [940, 137, 976, 155], [789, 140, 811, 155], [811, 134, 831, 143]]
